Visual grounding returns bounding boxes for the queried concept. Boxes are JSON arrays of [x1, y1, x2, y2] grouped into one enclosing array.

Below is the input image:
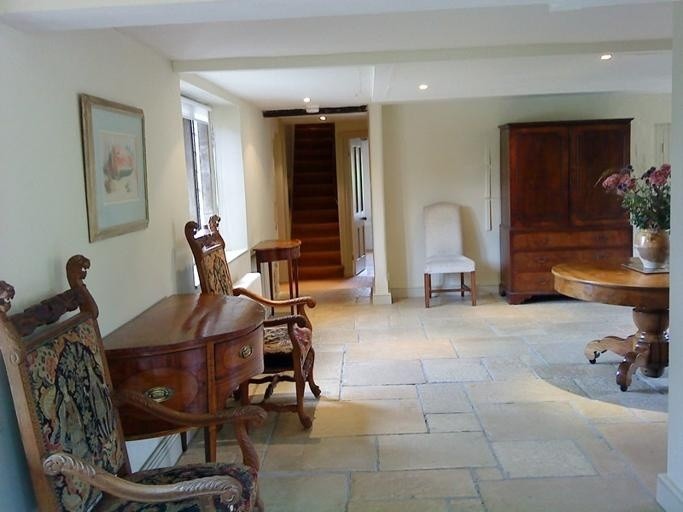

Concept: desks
[[101, 291, 266, 463], [551, 257, 670, 392], [253, 239, 302, 315]]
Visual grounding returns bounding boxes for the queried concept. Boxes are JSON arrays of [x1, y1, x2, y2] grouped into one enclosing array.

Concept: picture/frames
[[79, 93, 150, 243]]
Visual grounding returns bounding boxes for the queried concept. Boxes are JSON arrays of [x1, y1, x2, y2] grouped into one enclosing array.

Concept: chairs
[[424, 202, 477, 308], [0, 254, 267, 512], [184, 214, 321, 428]]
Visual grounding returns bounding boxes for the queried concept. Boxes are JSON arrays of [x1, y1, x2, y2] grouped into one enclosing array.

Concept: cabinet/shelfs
[[498, 117, 633, 305]]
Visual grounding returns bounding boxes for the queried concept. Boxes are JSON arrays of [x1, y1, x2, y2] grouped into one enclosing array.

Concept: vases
[[635, 228, 669, 269]]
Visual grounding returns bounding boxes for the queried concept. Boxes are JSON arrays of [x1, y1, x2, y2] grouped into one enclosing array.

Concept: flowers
[[601, 161, 671, 230]]
